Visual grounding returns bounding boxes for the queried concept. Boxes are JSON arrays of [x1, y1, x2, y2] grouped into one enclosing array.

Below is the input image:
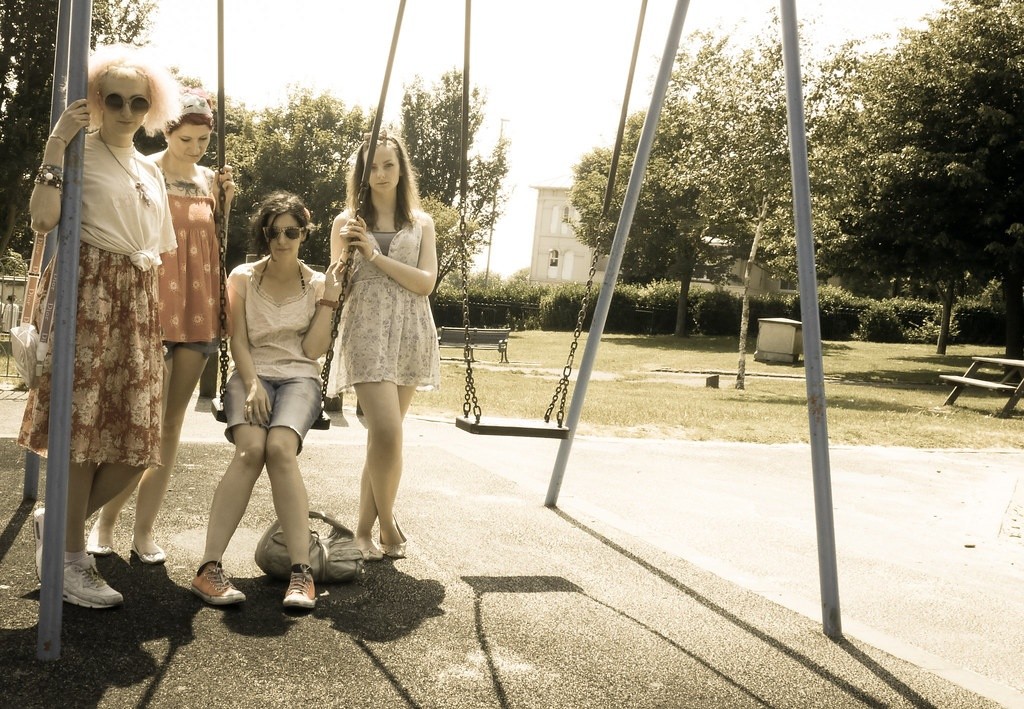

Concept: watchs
[[369, 249, 380, 263]]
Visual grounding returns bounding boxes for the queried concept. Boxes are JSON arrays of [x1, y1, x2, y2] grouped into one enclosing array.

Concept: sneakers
[[282, 564, 318, 608], [62, 554, 123, 609], [191, 560, 246, 604], [33, 508, 46, 582]]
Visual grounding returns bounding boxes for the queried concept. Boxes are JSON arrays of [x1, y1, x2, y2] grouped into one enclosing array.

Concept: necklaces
[[98, 129, 151, 207]]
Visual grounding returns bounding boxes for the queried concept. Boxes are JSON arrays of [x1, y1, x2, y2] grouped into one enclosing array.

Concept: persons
[[193, 193, 346, 609], [331, 135, 439, 562], [15, 51, 176, 606], [87, 96, 238, 563], [2, 295, 19, 333]]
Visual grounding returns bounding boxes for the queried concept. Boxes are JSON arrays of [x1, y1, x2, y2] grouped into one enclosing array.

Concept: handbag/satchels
[[254, 509, 364, 582], [9, 325, 41, 389]]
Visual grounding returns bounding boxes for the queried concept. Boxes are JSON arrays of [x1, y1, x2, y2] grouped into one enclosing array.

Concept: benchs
[[436, 326, 511, 362], [939, 374, 1017, 392]]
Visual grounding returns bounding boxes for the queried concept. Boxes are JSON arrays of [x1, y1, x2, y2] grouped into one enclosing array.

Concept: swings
[[211, 0, 406, 432], [455, 0, 650, 440]]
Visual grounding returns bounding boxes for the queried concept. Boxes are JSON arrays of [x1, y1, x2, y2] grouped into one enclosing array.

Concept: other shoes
[[132, 534, 166, 565], [377, 512, 408, 558], [355, 537, 383, 561], [87, 519, 114, 556]]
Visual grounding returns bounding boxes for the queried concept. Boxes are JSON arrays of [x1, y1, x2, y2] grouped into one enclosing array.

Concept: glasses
[[103, 92, 151, 116], [263, 226, 305, 241]]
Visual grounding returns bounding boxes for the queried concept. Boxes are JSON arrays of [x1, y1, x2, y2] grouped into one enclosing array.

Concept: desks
[[940, 357, 1024, 420]]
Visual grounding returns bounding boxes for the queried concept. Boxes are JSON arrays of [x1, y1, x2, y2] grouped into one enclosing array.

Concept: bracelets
[[48, 135, 68, 146], [320, 298, 339, 309], [34, 162, 63, 189]]
